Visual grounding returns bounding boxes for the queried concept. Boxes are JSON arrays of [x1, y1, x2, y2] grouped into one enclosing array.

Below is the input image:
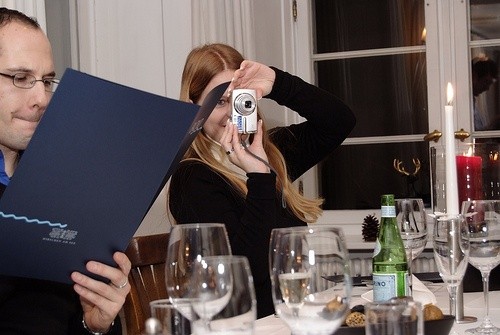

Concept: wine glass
[[165, 223, 234, 335], [459, 200, 500, 335], [278, 233, 311, 335], [433, 214, 474, 335]]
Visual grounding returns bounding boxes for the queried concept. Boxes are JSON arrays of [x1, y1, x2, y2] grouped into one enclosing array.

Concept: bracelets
[[82, 314, 114, 335]]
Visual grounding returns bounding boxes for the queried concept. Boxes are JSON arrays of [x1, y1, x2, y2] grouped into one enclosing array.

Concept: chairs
[[121, 233, 174, 335]]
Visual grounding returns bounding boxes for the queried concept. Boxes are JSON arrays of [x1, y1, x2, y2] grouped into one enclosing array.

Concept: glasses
[[0, 72, 61, 93]]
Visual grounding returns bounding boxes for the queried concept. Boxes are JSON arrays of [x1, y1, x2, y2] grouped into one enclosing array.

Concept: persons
[[472, 56, 500, 131], [167, 43, 357, 320], [0, 7, 131, 335]]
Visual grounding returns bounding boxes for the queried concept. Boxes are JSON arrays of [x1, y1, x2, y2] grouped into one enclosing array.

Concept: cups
[[394, 198, 428, 260], [432, 142, 487, 233], [191, 254, 257, 335], [365, 299, 423, 335], [270, 223, 352, 334], [149, 299, 207, 335]]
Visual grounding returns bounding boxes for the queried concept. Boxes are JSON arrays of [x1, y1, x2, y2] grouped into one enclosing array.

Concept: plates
[[303, 292, 346, 304]]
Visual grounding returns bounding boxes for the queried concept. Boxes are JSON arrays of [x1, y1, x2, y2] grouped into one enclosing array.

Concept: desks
[[197, 279, 500, 335]]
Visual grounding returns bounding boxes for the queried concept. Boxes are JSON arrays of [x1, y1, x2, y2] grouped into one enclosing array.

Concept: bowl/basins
[[334, 315, 455, 335]]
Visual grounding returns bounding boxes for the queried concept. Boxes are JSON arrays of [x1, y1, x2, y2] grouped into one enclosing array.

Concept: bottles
[[371, 195, 412, 303]]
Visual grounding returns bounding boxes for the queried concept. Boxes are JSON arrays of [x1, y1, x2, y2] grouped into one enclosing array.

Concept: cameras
[[231, 88, 258, 134]]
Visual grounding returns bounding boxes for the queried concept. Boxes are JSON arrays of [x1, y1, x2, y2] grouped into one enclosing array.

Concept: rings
[[226, 149, 233, 155], [112, 281, 127, 288]]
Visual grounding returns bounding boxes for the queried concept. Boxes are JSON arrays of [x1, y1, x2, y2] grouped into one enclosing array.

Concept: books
[[0, 68, 231, 285]]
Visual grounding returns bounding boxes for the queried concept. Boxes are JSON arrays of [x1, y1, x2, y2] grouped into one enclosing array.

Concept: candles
[[443, 80, 462, 217], [456, 146, 482, 222]]
